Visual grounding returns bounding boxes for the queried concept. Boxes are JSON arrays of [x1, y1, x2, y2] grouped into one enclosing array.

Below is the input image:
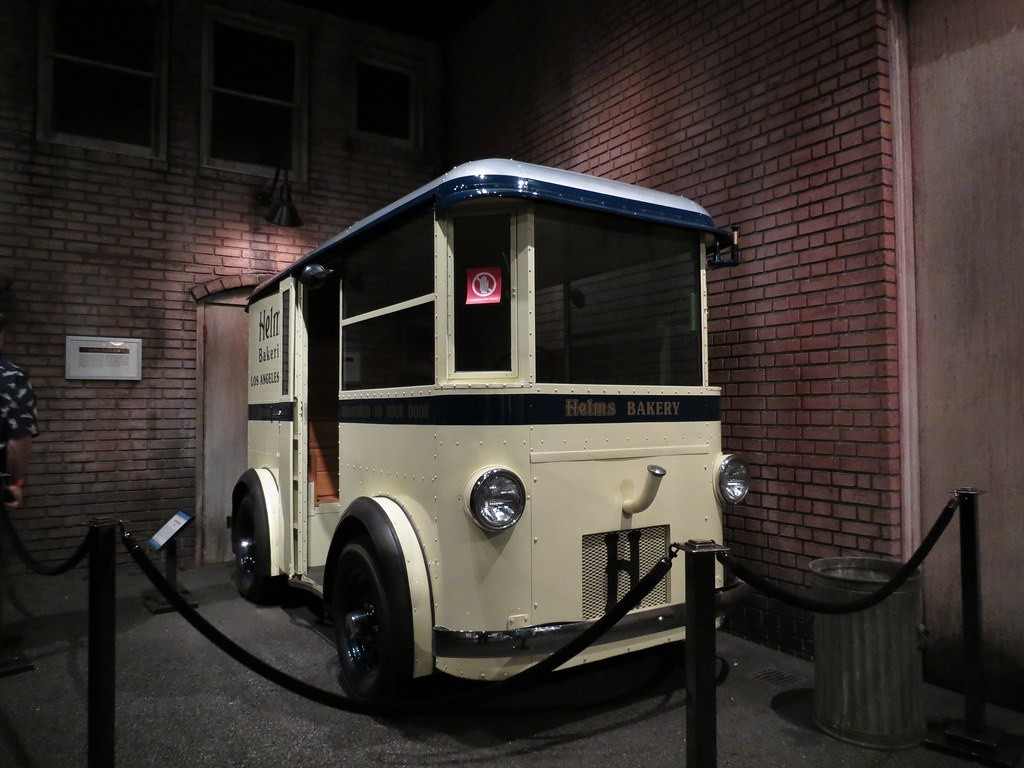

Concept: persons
[[0, 280, 39, 653]]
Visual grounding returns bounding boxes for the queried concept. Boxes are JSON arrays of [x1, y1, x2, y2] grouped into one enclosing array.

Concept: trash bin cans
[[811, 557, 928, 750]]
